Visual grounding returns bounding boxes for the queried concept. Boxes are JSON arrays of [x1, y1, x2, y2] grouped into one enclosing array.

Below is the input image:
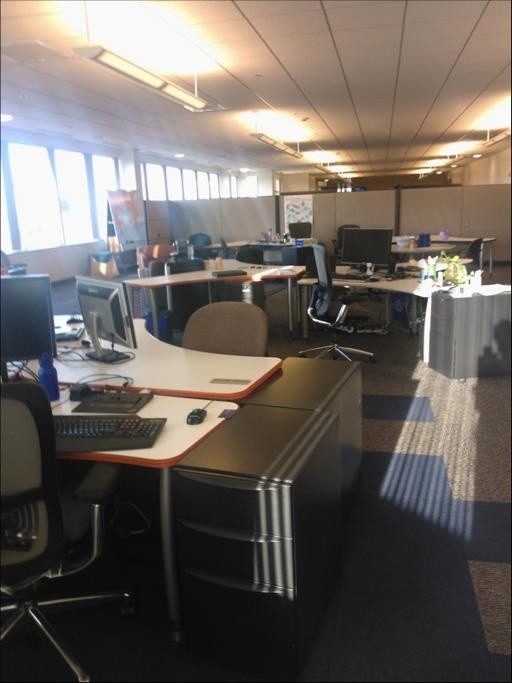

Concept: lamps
[[442, 150, 464, 167], [71, 0, 209, 110], [482, 122, 511, 148], [311, 153, 346, 180], [247, 108, 304, 158]]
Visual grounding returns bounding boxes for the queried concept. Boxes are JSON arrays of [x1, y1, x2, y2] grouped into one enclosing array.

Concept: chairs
[[186, 235, 318, 310], [10, 380, 133, 683], [181, 301, 268, 356], [298, 243, 387, 368]]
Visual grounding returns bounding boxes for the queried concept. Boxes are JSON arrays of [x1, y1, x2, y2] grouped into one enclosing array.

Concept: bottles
[[187, 243, 194, 260], [439, 227, 449, 240], [396, 232, 431, 248], [367, 263, 374, 276], [267, 229, 287, 243], [38, 351, 59, 400], [203, 256, 224, 269]]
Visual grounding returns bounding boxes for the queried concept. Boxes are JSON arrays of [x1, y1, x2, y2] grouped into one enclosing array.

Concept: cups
[[422, 262, 481, 287], [169, 237, 190, 257]]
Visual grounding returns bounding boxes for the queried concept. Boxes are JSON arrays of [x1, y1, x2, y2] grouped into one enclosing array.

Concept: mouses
[[367, 274, 380, 282], [185, 408, 206, 425], [66, 316, 82, 324]]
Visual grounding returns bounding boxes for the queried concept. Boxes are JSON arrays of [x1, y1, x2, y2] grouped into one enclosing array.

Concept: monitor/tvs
[[340, 227, 393, 274], [288, 221, 312, 240], [74, 274, 138, 364], [0, 273, 58, 381]]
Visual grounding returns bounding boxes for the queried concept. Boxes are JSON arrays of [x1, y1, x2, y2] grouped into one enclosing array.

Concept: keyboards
[[52, 414, 167, 452], [56, 328, 84, 341], [333, 273, 370, 280]]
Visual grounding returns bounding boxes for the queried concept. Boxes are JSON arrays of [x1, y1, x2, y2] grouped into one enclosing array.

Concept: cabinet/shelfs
[[145, 200, 171, 243], [241, 358, 363, 521], [177, 405, 340, 613], [430, 287, 510, 378]]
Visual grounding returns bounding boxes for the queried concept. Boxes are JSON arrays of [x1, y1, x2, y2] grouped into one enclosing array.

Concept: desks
[[11, 315, 279, 640], [118, 259, 305, 339], [390, 236, 493, 278], [298, 274, 470, 354]]
